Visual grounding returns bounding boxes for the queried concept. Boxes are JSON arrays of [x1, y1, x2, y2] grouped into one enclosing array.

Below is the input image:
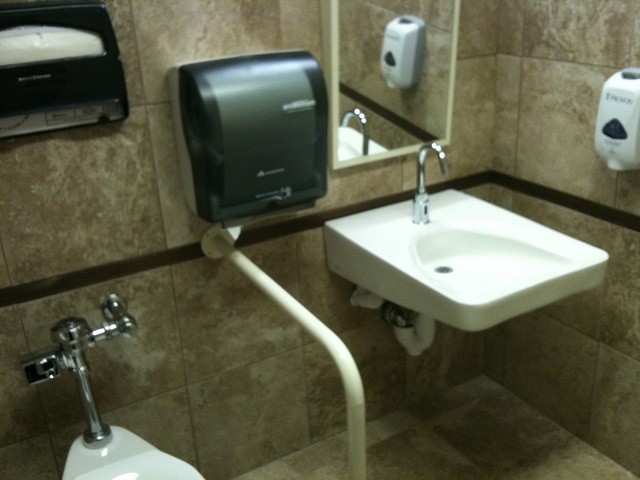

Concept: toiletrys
[[593, 67, 640, 173], [379, 14, 425, 90]]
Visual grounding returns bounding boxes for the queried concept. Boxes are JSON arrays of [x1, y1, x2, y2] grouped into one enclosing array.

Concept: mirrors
[[322, 0, 463, 171]]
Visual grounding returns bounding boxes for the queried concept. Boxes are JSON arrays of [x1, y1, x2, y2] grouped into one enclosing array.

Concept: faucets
[[340, 107, 370, 155], [411, 141, 449, 226]]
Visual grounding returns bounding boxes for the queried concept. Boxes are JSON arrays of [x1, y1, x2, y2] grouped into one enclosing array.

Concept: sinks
[[338, 126, 388, 162], [323, 187, 609, 333]]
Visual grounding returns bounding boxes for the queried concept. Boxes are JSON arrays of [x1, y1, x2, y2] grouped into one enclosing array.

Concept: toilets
[[60, 425, 207, 480]]
